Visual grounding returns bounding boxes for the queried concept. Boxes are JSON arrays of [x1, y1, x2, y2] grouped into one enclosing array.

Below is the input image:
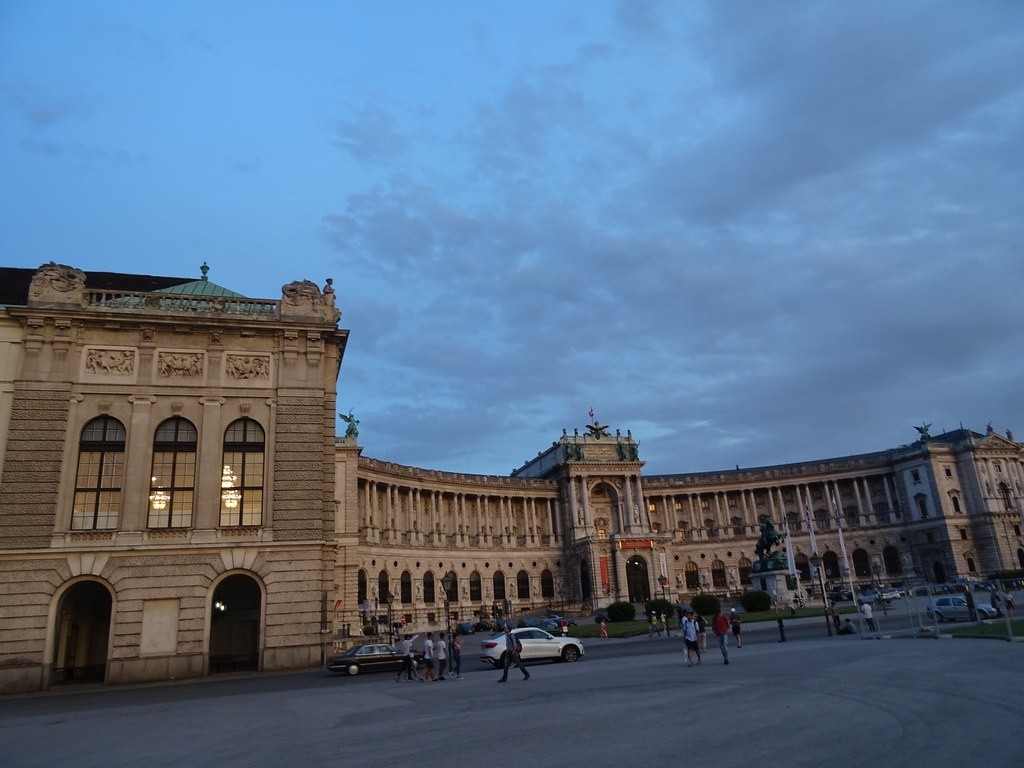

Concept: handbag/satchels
[[422, 651, 425, 658], [683, 645, 688, 663]]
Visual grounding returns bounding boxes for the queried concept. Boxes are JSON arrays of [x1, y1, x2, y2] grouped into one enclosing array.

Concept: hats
[[652, 611, 656, 613], [731, 608, 736, 612]]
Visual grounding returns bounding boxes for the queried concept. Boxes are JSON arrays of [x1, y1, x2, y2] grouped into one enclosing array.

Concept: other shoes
[[394, 675, 400, 682], [523, 673, 529, 680], [408, 678, 415, 681], [724, 659, 729, 665], [448, 672, 454, 679], [737, 645, 742, 648], [432, 677, 438, 681], [456, 674, 465, 679], [688, 662, 692, 667], [439, 676, 446, 680], [697, 659, 701, 664], [498, 679, 506, 682]]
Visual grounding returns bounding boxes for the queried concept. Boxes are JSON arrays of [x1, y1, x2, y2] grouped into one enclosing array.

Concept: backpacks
[[510, 634, 522, 652], [648, 615, 652, 623]]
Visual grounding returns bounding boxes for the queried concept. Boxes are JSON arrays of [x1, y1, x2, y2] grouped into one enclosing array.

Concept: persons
[[860, 601, 876, 631], [346, 410, 360, 438], [566, 441, 640, 461], [760, 517, 768, 547], [600, 619, 608, 640], [393, 631, 464, 681], [649, 607, 742, 667], [323, 279, 336, 307], [837, 618, 857, 634], [370, 569, 739, 596], [87, 350, 270, 381], [991, 584, 1016, 619], [831, 601, 841, 632], [497, 625, 530, 683]]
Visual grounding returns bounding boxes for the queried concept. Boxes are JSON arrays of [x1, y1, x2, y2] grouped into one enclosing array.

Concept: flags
[[589, 409, 594, 416]]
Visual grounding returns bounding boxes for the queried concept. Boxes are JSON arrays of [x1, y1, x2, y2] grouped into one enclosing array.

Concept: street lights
[[558, 588, 566, 619], [441, 571, 454, 672], [872, 562, 887, 615], [658, 575, 670, 636], [386, 591, 395, 646], [812, 550, 832, 636]]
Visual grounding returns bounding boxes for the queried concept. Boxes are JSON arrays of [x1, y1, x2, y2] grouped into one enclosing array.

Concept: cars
[[831, 579, 966, 606], [671, 604, 694, 619], [479, 627, 585, 670], [473, 621, 491, 632], [456, 623, 476, 635], [926, 596, 997, 623], [326, 643, 425, 676], [497, 615, 577, 632]]
